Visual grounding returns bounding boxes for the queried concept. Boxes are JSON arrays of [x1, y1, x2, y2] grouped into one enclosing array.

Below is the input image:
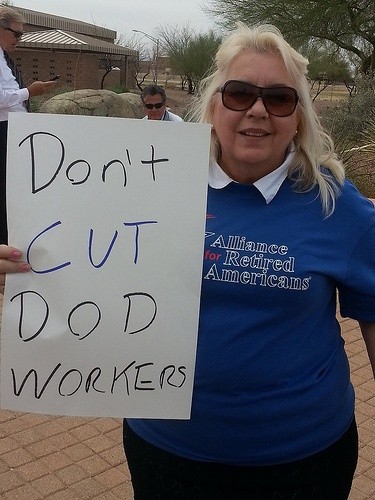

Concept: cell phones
[[50, 75, 60, 81]]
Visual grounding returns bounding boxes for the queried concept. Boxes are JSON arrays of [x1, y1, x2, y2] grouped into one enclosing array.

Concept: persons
[[0, 5, 57, 244], [137, 84, 184, 123], [0, 20, 375, 500]]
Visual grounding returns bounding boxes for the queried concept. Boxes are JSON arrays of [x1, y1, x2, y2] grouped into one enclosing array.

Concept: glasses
[[4, 27, 25, 38], [218, 79, 299, 117], [142, 98, 164, 109]]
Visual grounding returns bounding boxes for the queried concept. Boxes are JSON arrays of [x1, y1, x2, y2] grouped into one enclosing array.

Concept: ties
[[3, 51, 32, 112]]
